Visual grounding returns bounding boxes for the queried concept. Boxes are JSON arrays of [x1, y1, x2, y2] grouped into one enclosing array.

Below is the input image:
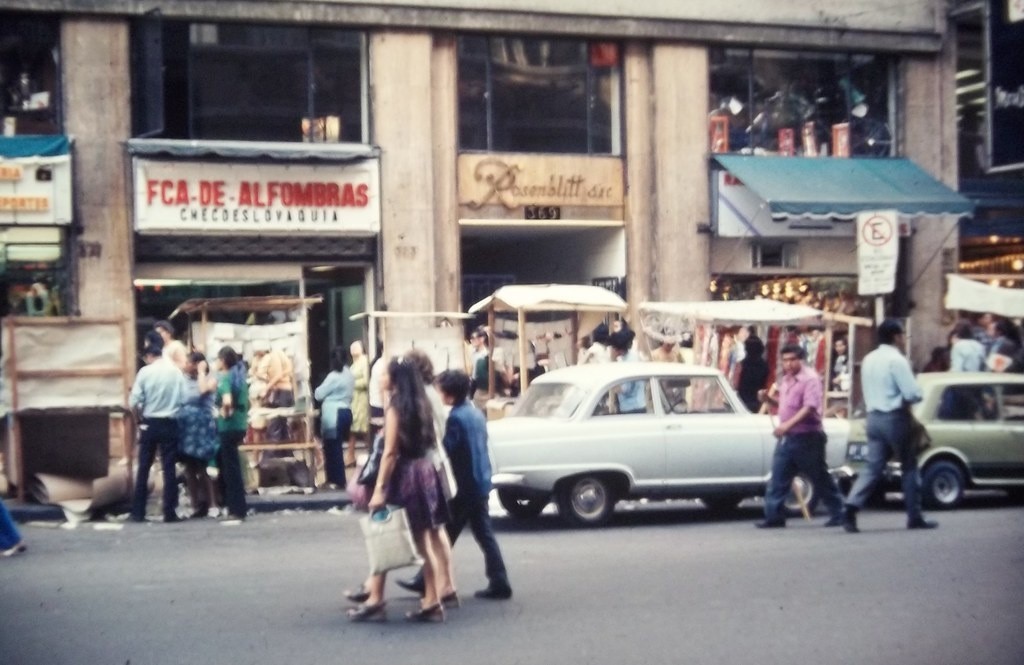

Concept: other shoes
[[344, 584, 370, 602], [754, 520, 785, 528], [0, 540, 26, 557], [841, 508, 860, 533], [824, 518, 842, 527], [125, 514, 145, 522], [163, 515, 182, 522], [907, 518, 938, 529]]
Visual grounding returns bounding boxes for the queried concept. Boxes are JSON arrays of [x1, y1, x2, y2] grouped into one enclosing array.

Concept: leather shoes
[[395, 575, 425, 596], [440, 591, 459, 608], [475, 588, 512, 598]]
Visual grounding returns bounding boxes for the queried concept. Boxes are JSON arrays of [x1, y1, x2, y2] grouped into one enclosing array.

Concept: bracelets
[[375, 483, 387, 489]]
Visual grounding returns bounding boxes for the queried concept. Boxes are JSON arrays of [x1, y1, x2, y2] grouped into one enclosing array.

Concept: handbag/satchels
[[436, 458, 458, 499], [357, 435, 396, 491], [359, 504, 425, 576]]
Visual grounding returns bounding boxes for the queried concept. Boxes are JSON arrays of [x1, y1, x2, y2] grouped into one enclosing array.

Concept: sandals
[[406, 603, 443, 623], [347, 601, 386, 622]]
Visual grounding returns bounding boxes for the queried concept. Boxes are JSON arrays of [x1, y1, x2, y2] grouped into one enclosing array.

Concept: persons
[[0, 308, 1024, 625]]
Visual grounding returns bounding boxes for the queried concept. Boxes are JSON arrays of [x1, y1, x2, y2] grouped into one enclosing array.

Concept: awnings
[[710, 154, 974, 294]]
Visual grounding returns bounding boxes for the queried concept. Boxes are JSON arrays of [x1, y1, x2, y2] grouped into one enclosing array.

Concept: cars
[[854, 372, 1024, 511], [486, 361, 853, 529]]
